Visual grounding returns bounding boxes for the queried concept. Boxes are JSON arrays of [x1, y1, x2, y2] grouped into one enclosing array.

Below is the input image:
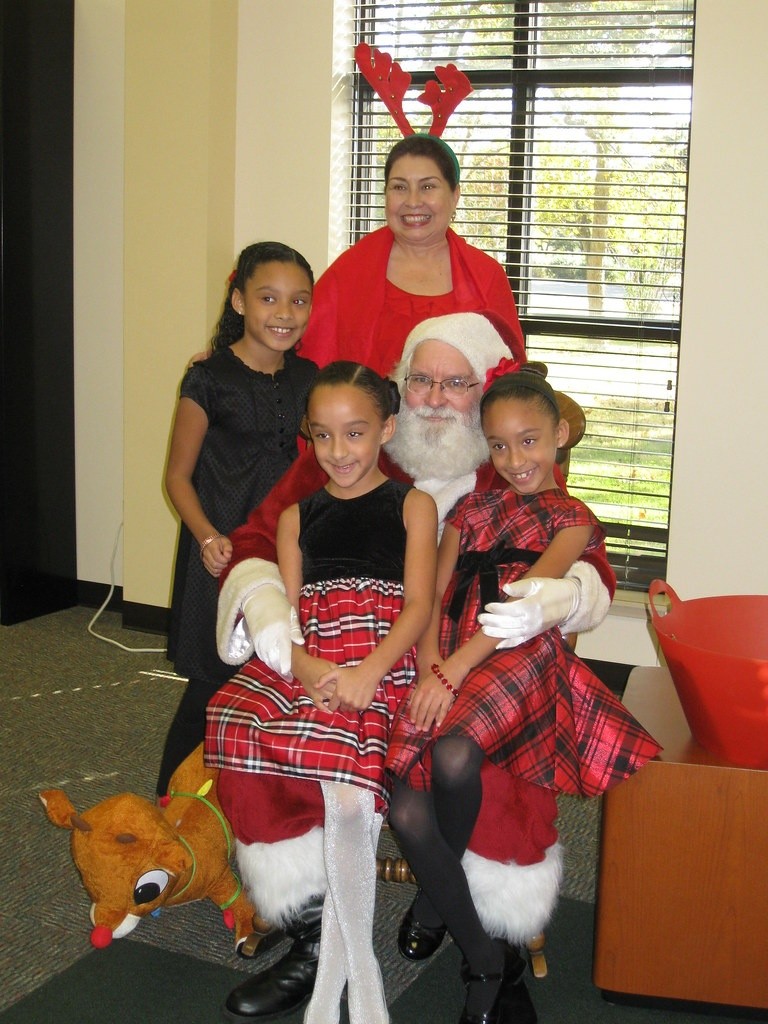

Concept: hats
[[400, 308, 527, 387]]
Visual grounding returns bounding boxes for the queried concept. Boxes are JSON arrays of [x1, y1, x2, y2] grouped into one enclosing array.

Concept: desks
[[593, 667, 768, 1019]]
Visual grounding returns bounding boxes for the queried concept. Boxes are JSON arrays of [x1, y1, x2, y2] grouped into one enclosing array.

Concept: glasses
[[405, 372, 481, 394]]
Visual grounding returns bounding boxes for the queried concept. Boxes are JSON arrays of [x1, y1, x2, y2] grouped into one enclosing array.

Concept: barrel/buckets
[[647, 577, 768, 771]]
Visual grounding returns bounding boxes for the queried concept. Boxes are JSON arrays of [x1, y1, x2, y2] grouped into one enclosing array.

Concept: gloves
[[476, 575, 583, 651], [240, 583, 307, 683]]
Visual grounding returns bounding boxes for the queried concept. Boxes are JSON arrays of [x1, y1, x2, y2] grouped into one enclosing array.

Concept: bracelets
[[199, 534, 225, 564], [431, 664, 460, 697]]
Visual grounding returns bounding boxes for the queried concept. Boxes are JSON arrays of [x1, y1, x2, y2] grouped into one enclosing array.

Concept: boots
[[460, 958, 539, 1023], [222, 895, 325, 1022]]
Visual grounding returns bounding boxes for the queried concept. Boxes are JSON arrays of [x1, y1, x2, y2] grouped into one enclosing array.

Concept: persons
[[379, 362, 665, 1024], [155, 241, 320, 803], [184, 134, 527, 456], [200, 359, 438, 1024], [214, 310, 618, 1024]]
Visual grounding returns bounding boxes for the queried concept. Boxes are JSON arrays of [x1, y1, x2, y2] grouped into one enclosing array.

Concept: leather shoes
[[394, 885, 448, 964], [459, 938, 528, 1023]]
